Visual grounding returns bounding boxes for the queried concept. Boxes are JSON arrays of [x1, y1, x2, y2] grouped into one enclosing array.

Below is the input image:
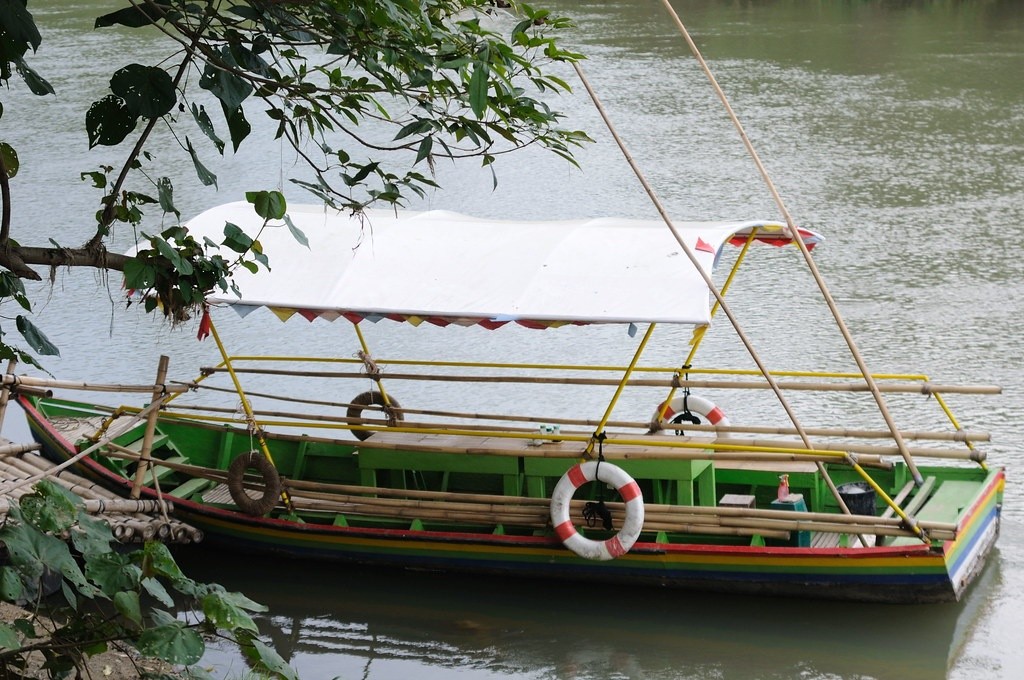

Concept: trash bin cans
[[835, 481, 877, 517]]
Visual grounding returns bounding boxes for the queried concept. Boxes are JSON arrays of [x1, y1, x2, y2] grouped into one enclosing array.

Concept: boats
[[13, 199, 1006, 604]]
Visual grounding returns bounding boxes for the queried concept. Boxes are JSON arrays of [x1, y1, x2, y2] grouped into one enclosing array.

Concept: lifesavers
[[224, 453, 281, 516], [345, 391, 408, 445], [651, 394, 732, 449], [548, 458, 647, 561]]
